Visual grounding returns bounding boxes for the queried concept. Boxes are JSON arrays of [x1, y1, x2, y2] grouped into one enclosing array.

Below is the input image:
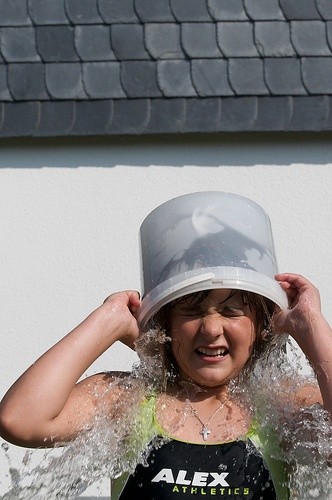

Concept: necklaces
[[183, 392, 232, 441]]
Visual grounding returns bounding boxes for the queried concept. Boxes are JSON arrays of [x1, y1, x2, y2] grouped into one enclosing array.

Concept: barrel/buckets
[[134, 191, 288, 347]]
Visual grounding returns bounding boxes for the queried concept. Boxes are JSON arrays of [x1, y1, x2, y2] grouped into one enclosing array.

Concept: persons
[[0, 191, 332, 500]]
